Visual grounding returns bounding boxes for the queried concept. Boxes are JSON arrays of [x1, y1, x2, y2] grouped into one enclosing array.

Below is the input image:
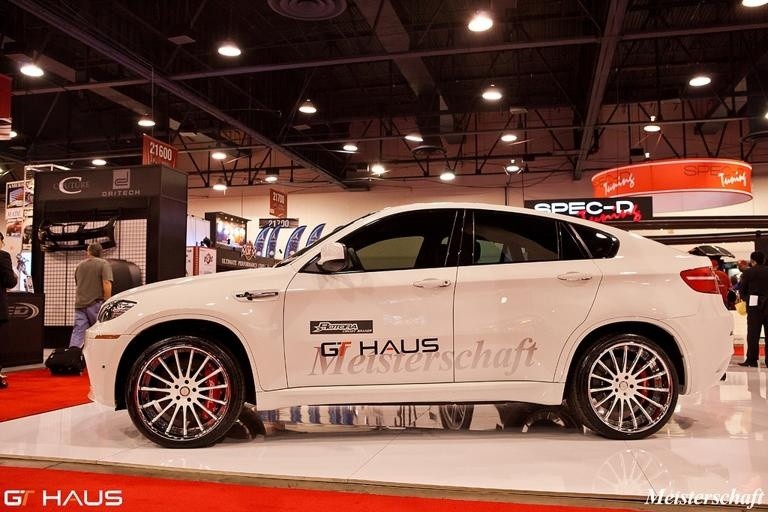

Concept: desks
[[0, 292, 46, 368]]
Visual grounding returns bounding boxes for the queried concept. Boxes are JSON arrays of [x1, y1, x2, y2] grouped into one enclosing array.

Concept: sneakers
[[737, 357, 758, 366]]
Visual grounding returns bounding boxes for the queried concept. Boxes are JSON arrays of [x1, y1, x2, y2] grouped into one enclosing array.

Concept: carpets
[[0, 365, 95, 424]]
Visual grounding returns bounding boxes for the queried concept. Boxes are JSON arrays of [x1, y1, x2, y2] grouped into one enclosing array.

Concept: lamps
[[137, 111, 156, 127], [500, 130, 517, 142], [211, 142, 228, 191], [297, 98, 318, 114], [506, 158, 520, 173], [201, 236, 213, 248], [217, 1, 243, 58], [440, 168, 456, 180], [404, 121, 427, 143], [342, 142, 359, 152], [480, 81, 502, 100]]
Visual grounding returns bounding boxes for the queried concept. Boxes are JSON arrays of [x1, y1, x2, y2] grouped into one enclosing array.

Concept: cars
[[81, 201, 734, 449]]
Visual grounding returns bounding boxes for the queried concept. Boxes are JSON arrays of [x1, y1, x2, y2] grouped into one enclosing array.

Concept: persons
[[69, 242, 114, 349], [733, 252, 768, 368], [6, 262, 29, 292], [0, 232, 17, 388]]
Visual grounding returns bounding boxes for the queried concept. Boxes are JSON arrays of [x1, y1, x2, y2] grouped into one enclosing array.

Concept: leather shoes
[[1, 374, 7, 386]]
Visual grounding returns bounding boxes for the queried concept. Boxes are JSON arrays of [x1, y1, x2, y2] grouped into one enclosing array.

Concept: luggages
[[45, 343, 86, 376]]
